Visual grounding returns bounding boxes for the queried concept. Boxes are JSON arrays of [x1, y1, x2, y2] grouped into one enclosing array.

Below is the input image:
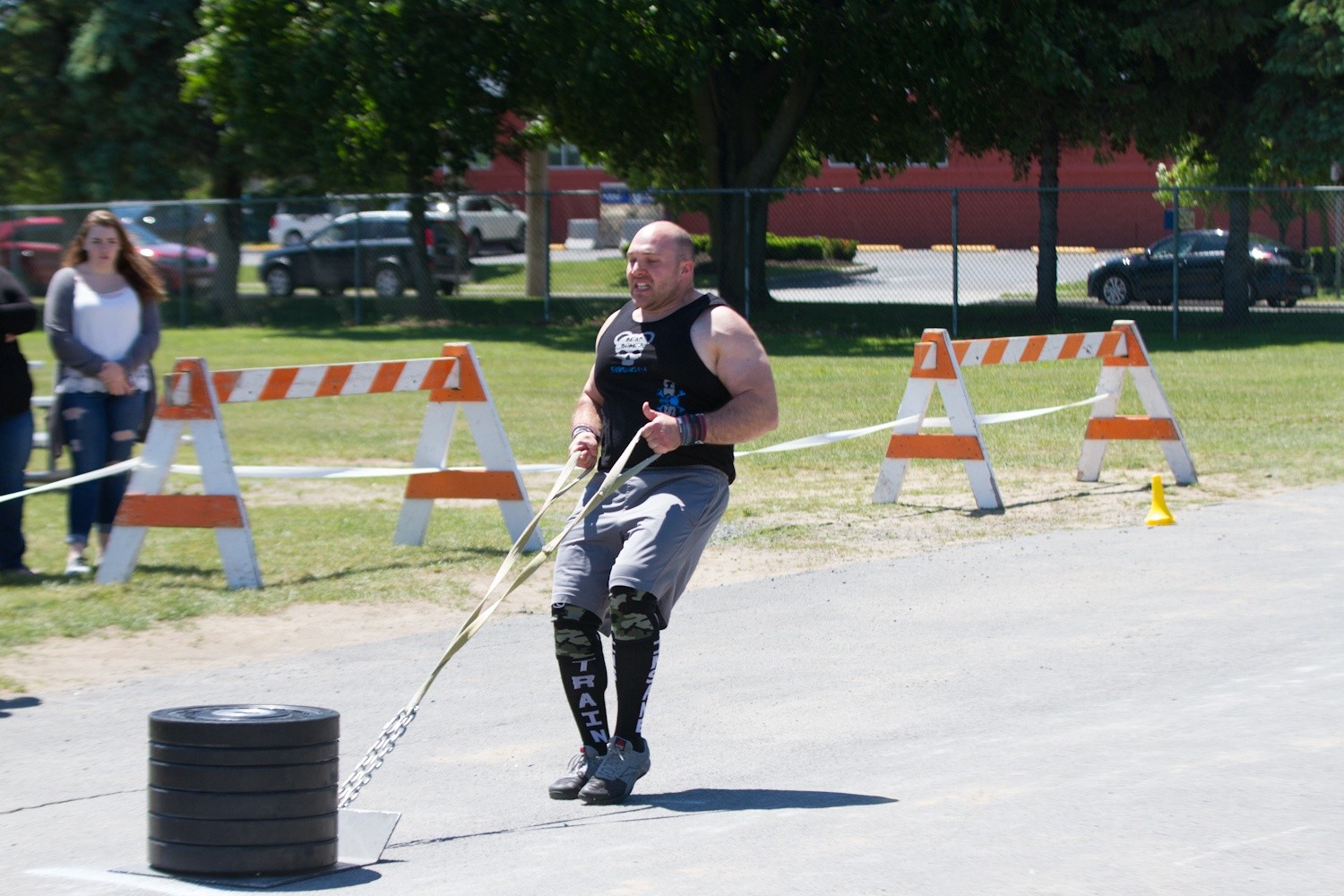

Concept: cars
[[107, 195, 221, 252], [1085, 226, 1319, 309], [1, 213, 220, 299], [263, 195, 362, 248]]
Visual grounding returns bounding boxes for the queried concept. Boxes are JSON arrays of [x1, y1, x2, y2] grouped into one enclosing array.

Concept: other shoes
[[0, 566, 43, 583], [64, 557, 90, 578]]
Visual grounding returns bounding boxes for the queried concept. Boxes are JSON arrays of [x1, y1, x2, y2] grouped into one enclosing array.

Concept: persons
[[43, 211, 163, 574], [0, 266, 41, 585], [548, 220, 778, 804]]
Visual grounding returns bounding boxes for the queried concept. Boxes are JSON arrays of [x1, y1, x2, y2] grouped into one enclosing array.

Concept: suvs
[[383, 192, 528, 258], [259, 207, 477, 303]]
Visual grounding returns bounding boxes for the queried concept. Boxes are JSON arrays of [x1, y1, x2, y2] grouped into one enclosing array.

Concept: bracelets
[[677, 413, 706, 446], [571, 424, 600, 443]]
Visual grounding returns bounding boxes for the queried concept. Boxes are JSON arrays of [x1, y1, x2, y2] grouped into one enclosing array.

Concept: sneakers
[[578, 736, 650, 804], [549, 746, 609, 799]]
